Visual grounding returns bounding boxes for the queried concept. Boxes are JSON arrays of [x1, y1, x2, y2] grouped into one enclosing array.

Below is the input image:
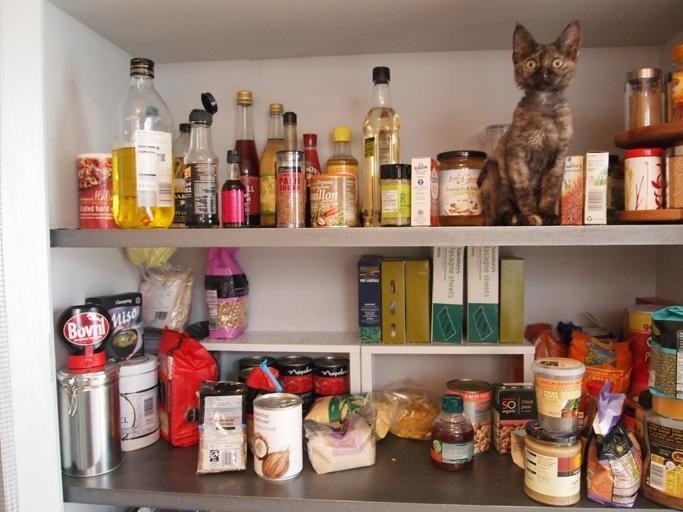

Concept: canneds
[[380, 164, 412, 227], [308, 173, 359, 228], [436, 149, 490, 226], [525, 420, 584, 505], [623, 67, 683, 211], [238, 356, 349, 482], [446, 379, 492, 457], [75, 152, 121, 229], [626, 304, 671, 403]]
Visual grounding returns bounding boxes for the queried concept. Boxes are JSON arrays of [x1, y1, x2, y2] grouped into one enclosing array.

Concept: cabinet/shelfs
[[41, 1, 683, 511]]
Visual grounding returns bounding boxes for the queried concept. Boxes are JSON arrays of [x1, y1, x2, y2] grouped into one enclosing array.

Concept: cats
[[477, 22, 582, 225]]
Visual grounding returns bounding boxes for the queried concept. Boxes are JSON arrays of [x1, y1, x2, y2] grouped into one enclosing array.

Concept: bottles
[[428, 393, 476, 472], [360, 65, 404, 227], [175, 87, 357, 229], [483, 124, 512, 158], [55, 369, 123, 479], [110, 56, 175, 232]]
